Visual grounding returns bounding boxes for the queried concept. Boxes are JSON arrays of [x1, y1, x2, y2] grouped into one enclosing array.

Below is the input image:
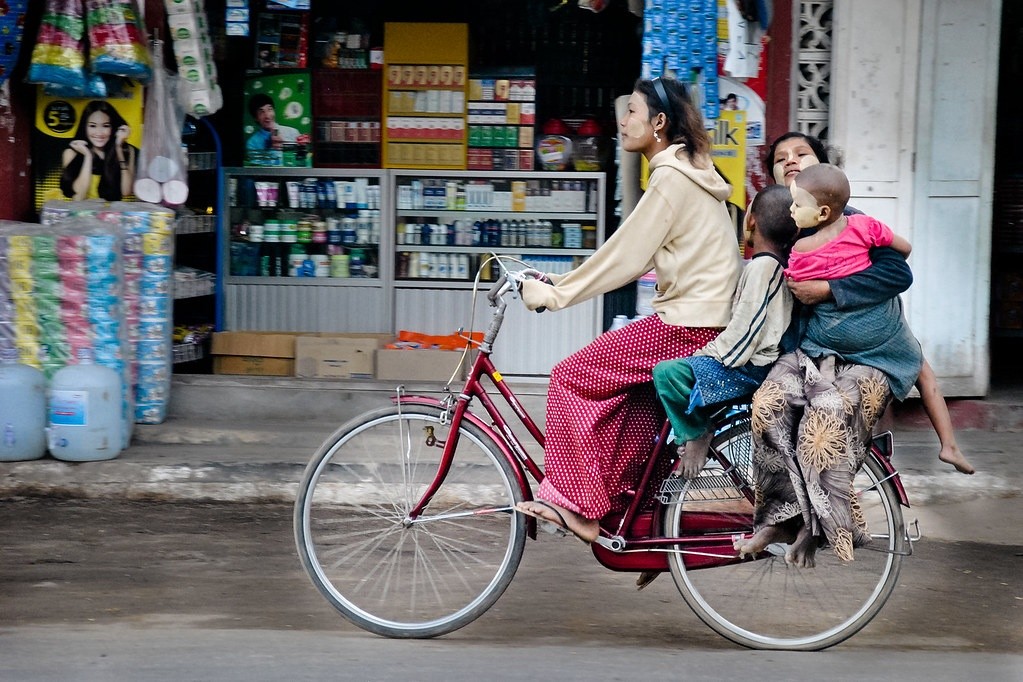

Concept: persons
[[60, 101, 140, 202], [513, 76, 743, 547], [246, 94, 301, 166], [726, 93, 739, 110], [734, 132, 913, 568], [783, 163, 975, 474], [652, 185, 799, 478]]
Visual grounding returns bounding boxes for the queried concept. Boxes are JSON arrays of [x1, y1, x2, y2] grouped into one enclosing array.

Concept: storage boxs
[[296, 332, 480, 382], [210, 330, 299, 378]]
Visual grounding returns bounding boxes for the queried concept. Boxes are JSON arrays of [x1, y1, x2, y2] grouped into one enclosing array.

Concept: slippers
[[637, 567, 662, 591], [514, 500, 590, 548]]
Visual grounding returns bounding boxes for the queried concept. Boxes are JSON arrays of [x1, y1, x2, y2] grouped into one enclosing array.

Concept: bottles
[[248, 178, 598, 278], [0, 350, 47, 462], [49, 350, 122, 462]]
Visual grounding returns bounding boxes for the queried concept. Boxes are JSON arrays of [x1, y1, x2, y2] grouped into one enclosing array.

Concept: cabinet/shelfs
[[224, 166, 391, 333], [391, 169, 606, 375], [245, 6, 539, 168], [169, 117, 225, 364]]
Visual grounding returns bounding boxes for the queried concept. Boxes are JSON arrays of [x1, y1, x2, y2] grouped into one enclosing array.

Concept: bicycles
[[289, 251, 922, 652]]
